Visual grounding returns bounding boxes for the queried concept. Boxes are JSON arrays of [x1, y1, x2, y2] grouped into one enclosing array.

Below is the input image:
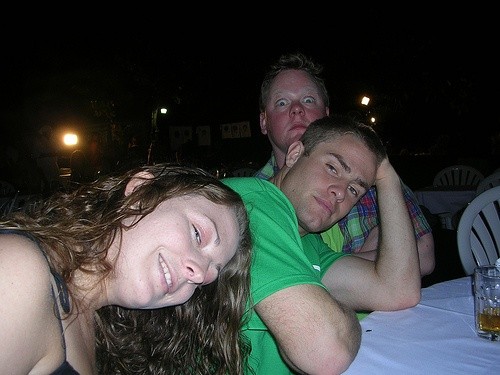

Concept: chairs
[[433, 164, 484, 189], [477, 172, 499, 194], [456, 186, 500, 275]]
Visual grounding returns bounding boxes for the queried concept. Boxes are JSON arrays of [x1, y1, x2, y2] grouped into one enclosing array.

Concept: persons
[[217, 107, 423, 375], [250, 51, 437, 278], [0, 159, 257, 375]]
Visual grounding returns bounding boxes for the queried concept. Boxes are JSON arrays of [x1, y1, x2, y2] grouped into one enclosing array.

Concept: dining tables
[[415, 183, 483, 231], [339, 272, 500, 375]]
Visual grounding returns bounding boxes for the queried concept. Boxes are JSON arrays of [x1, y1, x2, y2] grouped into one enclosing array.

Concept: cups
[[471, 265, 500, 341]]
